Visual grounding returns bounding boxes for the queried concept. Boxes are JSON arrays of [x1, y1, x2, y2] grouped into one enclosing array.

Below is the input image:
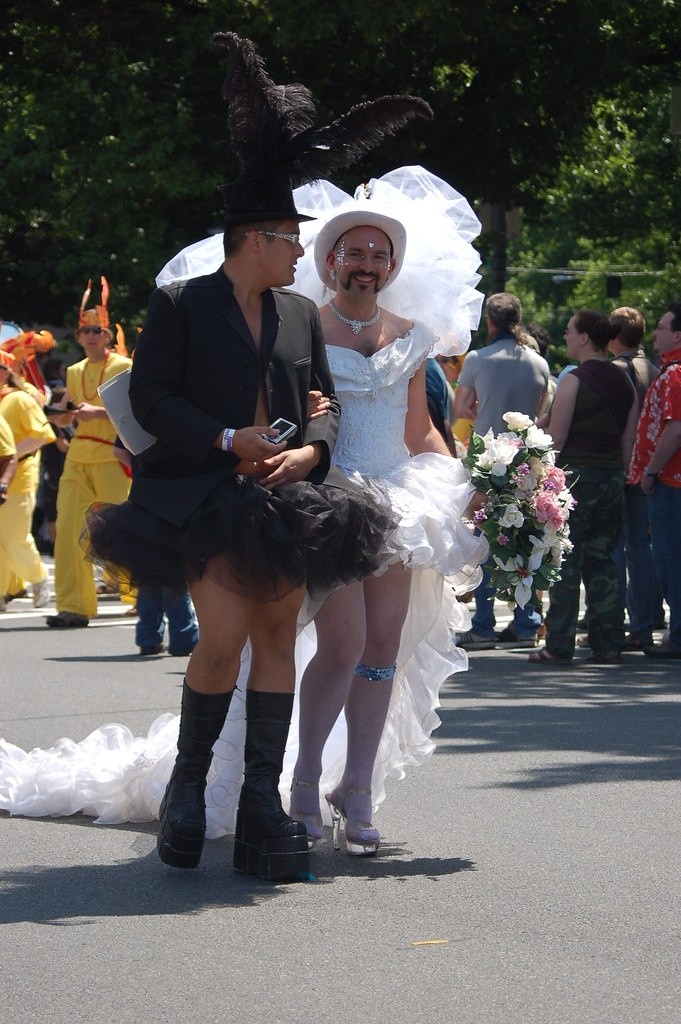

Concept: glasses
[[246, 230, 300, 244], [78, 327, 107, 335]]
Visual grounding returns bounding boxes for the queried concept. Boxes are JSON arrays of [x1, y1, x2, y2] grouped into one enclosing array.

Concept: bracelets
[[222, 428, 236, 452]]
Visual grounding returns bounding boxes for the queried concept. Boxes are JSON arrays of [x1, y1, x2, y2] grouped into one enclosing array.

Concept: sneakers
[[494, 621, 537, 649], [32, 563, 50, 608], [452, 631, 495, 649]]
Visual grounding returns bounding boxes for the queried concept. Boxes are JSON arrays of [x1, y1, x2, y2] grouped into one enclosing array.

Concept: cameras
[[260, 417, 297, 445]]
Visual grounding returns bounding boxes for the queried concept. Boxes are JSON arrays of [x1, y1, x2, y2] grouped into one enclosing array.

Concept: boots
[[233, 688, 309, 880], [156, 677, 240, 869]]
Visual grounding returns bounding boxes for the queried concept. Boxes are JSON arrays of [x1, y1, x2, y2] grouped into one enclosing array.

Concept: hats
[[78, 276, 110, 330], [212, 178, 317, 235], [313, 210, 407, 294]]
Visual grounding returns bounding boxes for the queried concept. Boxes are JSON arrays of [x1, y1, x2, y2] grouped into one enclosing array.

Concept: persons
[[82, 173, 379, 881], [0, 165, 487, 862], [0, 276, 204, 660], [430, 289, 681, 667]]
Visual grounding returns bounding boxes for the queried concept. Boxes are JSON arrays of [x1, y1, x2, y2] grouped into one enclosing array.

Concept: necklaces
[[328, 299, 380, 334], [79, 353, 113, 401]]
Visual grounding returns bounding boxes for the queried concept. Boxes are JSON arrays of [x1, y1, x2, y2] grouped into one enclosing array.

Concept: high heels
[[290, 778, 324, 852], [325, 788, 380, 854]]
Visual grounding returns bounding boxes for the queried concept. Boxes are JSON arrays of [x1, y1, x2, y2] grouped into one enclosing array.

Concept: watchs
[[642, 465, 660, 477]]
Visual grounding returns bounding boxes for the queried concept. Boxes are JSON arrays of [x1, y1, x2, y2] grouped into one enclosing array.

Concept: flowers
[[465, 412, 579, 614]]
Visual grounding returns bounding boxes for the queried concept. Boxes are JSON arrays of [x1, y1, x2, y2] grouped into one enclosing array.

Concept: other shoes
[[141, 643, 163, 654], [47, 612, 88, 627], [172, 639, 198, 656], [527, 647, 569, 665], [622, 635, 654, 651], [643, 643, 681, 657], [587, 650, 622, 664]]
